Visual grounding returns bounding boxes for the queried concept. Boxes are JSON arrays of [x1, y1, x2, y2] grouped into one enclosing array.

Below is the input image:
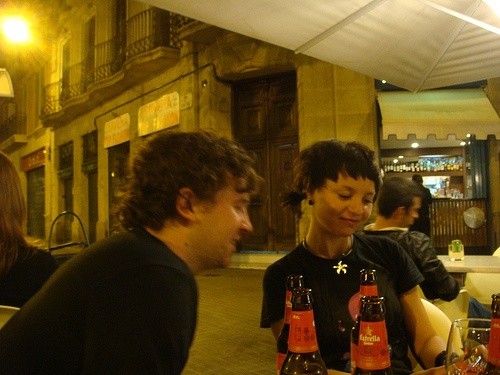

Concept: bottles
[[275, 268, 393, 375], [382, 159, 406, 173], [480, 293, 500, 375]]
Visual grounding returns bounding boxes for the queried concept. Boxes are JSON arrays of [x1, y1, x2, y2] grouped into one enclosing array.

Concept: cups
[[448, 245, 464, 261], [445, 318, 492, 375]]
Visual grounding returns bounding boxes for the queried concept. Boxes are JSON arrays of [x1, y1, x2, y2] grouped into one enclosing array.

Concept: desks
[[437, 254, 500, 290]]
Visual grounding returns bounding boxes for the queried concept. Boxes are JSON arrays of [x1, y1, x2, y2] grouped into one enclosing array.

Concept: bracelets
[[435, 350, 456, 367]]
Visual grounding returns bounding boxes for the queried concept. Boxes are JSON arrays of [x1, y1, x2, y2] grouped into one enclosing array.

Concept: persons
[[0, 130, 262, 375], [260, 139, 487, 375], [0, 151, 57, 309], [410, 174, 432, 238], [362, 175, 491, 319]]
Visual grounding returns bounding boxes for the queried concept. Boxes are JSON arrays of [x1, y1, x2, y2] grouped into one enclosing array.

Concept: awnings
[[377, 92, 500, 147]]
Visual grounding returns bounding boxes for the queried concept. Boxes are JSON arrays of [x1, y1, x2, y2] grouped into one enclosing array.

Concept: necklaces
[[303, 234, 354, 275]]
